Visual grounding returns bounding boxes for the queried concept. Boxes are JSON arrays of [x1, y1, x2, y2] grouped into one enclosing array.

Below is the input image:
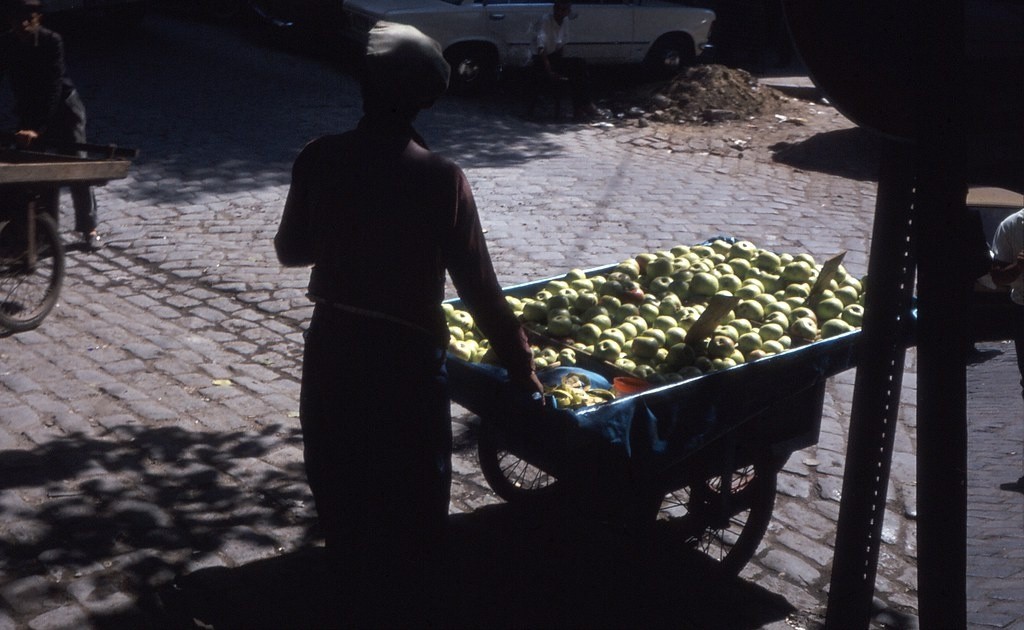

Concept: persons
[[274, 20, 543, 630], [988, 207, 1024, 374], [0, 0, 97, 256]]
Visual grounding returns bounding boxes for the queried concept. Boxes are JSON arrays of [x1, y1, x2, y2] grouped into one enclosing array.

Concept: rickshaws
[[0, 142, 127, 332], [430, 238, 919, 579]]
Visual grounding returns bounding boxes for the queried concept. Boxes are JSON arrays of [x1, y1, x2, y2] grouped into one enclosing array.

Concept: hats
[[366, 18, 451, 104]]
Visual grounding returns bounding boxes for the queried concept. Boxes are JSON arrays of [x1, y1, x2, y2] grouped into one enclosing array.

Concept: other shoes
[[574, 101, 603, 116]]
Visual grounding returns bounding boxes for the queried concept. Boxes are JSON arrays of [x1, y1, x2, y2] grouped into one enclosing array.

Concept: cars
[[350, 3, 714, 96]]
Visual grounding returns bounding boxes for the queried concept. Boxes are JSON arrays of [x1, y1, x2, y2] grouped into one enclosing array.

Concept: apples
[[440, 301, 498, 365], [502, 239, 868, 388]]
